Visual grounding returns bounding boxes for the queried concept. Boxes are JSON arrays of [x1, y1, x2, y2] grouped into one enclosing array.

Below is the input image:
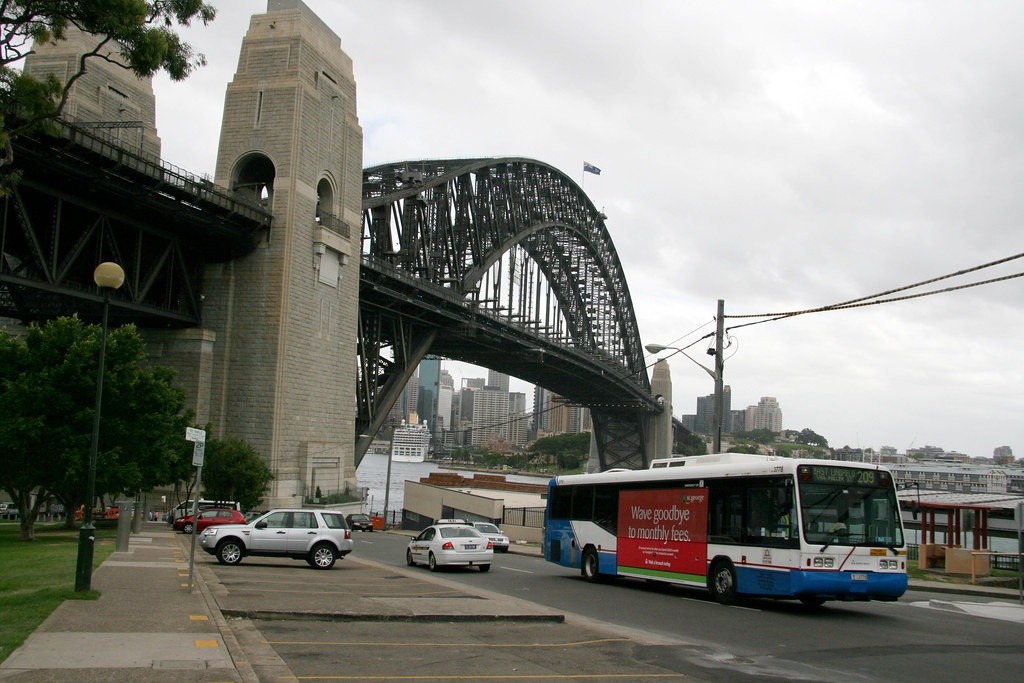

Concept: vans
[[164, 498, 241, 525]]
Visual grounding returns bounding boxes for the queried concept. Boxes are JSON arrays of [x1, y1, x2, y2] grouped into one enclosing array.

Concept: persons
[[776, 508, 793, 537], [149, 509, 159, 521]]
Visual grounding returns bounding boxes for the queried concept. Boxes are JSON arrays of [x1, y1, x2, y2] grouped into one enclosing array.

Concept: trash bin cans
[[371, 517, 384, 530]]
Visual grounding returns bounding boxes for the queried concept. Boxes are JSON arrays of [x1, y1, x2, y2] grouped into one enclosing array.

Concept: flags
[[583, 160, 601, 176]]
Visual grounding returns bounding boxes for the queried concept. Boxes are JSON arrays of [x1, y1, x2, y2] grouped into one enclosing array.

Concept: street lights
[[644, 299, 726, 454], [64, 261, 125, 598], [359, 433, 393, 529]]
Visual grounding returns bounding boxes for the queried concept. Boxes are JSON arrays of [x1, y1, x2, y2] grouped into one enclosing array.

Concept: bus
[[540, 453, 922, 611]]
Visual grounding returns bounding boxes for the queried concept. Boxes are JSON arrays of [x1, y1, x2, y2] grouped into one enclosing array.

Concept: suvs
[[199, 508, 355, 571]]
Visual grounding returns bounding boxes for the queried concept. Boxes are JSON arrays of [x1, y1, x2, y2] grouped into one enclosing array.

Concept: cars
[[345, 513, 374, 533], [407, 518, 494, 572], [468, 521, 510, 553], [244, 512, 262, 525], [175, 508, 246, 535]]
